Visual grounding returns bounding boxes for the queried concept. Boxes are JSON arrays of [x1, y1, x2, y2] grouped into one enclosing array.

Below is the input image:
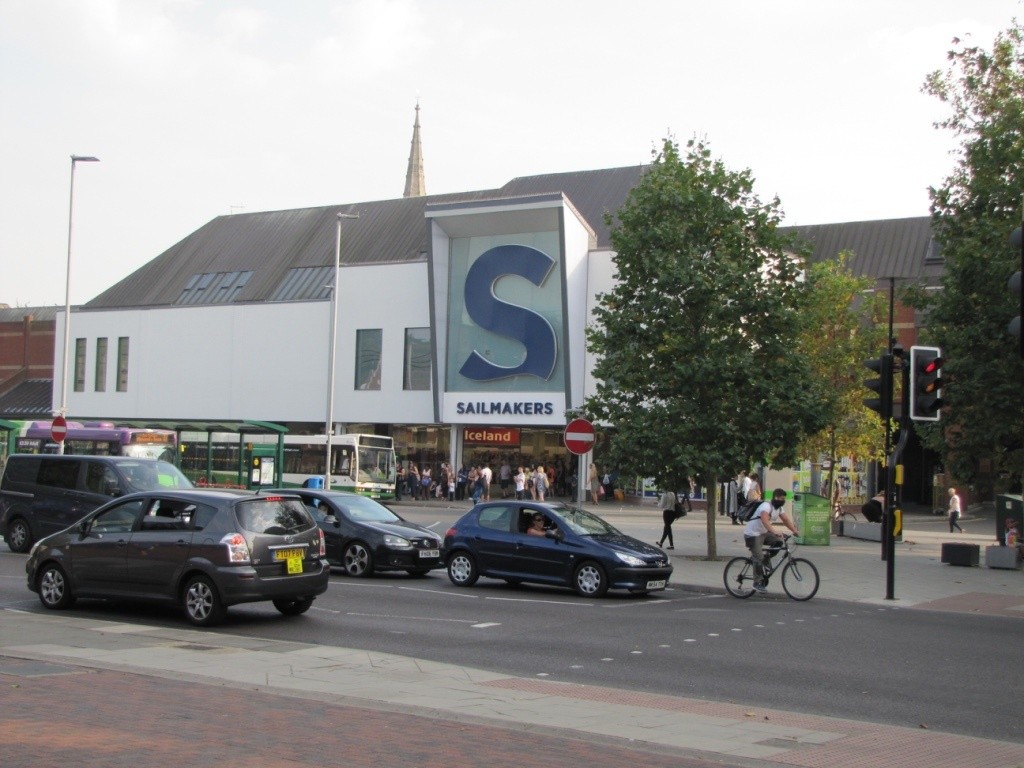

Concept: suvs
[[257, 488, 443, 579], [25, 488, 329, 626], [441, 499, 671, 596]]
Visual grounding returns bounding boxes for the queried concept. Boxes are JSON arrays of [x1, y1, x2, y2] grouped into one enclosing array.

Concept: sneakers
[[752, 581, 768, 593], [762, 560, 772, 571]]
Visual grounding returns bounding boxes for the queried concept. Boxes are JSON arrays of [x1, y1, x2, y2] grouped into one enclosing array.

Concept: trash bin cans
[[309, 478, 323, 508]]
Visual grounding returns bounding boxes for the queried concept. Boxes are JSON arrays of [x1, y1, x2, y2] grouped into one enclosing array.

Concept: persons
[[728, 477, 745, 526], [948, 488, 966, 533], [315, 502, 333, 523], [738, 469, 747, 503], [748, 474, 762, 501], [528, 513, 559, 536], [600, 465, 612, 502], [655, 490, 679, 549], [585, 464, 600, 504], [744, 488, 800, 594], [397, 449, 575, 506]]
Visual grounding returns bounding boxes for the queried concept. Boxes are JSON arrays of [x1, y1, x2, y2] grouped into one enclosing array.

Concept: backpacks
[[735, 500, 773, 522]]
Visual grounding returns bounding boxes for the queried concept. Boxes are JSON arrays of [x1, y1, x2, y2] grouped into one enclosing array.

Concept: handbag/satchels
[[543, 477, 550, 489], [435, 479, 444, 498], [747, 489, 759, 502], [597, 484, 605, 496], [675, 495, 687, 518]]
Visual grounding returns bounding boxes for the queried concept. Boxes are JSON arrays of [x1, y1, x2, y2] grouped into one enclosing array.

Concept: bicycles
[[829, 500, 859, 523], [724, 534, 820, 601]]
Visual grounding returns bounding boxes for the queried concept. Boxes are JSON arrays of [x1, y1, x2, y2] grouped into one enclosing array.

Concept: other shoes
[[656, 542, 662, 547], [666, 547, 674, 550]]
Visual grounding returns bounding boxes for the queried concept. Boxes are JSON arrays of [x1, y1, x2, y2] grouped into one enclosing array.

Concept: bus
[[180, 430, 398, 505], [1, 420, 177, 469]]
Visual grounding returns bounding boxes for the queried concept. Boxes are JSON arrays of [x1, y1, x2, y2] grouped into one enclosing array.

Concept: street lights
[[323, 212, 360, 488], [56, 156, 100, 452]]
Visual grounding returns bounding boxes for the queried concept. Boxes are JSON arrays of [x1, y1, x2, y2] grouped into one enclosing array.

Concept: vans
[[0, 454, 197, 554]]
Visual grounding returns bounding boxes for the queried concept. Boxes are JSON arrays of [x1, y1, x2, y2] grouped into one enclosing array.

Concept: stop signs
[[563, 418, 594, 455], [51, 417, 67, 443]]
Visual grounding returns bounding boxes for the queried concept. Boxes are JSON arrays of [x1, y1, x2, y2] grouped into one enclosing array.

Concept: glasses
[[534, 519, 544, 521]]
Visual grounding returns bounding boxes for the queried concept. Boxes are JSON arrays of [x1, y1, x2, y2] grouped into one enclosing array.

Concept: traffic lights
[[864, 352, 892, 424], [909, 346, 942, 422]]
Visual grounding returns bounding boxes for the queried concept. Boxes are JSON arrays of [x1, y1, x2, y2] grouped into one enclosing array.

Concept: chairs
[[521, 513, 532, 532], [156, 506, 175, 518]]
